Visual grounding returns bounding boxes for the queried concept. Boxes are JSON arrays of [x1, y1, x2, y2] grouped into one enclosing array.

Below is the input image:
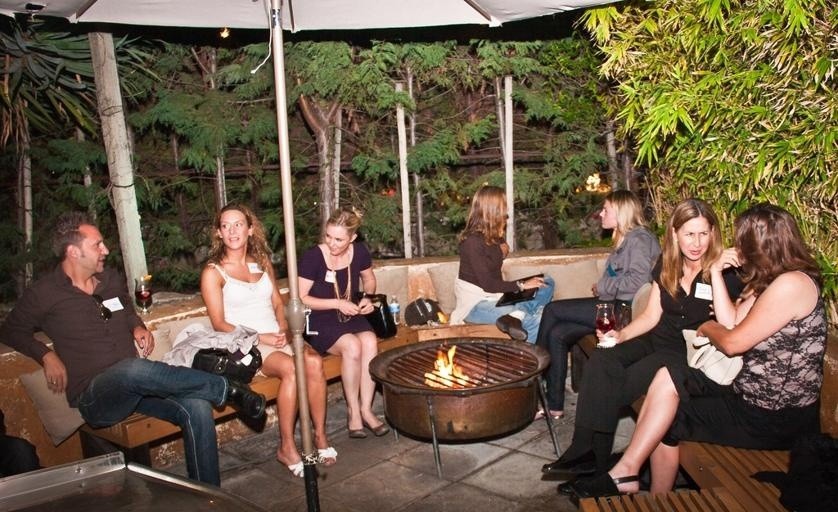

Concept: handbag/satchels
[[358, 292, 397, 338], [405, 298, 442, 326], [195, 346, 262, 383], [754, 433, 837, 512], [690, 334, 744, 386]]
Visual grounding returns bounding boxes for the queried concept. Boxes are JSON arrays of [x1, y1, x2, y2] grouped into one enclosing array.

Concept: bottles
[[389, 295, 400, 326]]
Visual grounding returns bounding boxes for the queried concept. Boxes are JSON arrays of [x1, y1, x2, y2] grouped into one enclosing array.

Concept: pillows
[[356, 266, 411, 325], [426, 261, 459, 317], [501, 257, 599, 303], [152, 316, 213, 349], [594, 258, 608, 275], [16, 328, 174, 450]]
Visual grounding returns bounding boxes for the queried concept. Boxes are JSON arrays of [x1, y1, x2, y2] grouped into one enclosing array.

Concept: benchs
[[74, 320, 420, 477], [570, 320, 838, 512], [403, 318, 515, 344]]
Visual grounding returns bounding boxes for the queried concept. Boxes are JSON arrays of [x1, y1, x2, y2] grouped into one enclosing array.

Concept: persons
[[542, 198, 747, 496], [297, 205, 389, 438], [533, 188, 662, 420], [0, 409, 41, 477], [201, 203, 338, 479], [0, 211, 266, 489], [570, 203, 827, 502], [450, 185, 555, 345]]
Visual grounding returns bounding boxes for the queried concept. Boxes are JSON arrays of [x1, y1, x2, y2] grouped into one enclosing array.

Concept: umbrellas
[[0, 0, 625, 512]]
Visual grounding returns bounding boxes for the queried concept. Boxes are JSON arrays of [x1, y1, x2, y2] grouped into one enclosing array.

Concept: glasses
[[91, 294, 112, 322]]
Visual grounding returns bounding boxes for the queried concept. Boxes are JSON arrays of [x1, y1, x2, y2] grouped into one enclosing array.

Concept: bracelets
[[520, 280, 524, 292]]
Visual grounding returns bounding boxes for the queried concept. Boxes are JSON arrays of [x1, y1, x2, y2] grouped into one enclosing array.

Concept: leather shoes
[[228, 380, 267, 420], [346, 417, 390, 438], [496, 315, 527, 341], [541, 452, 643, 503]]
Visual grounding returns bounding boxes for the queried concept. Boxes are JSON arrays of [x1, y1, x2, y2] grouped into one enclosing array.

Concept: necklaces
[[332, 265, 352, 323]]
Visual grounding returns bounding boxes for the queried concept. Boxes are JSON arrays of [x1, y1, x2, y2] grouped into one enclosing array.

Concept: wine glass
[[133, 277, 153, 316], [594, 303, 617, 348]]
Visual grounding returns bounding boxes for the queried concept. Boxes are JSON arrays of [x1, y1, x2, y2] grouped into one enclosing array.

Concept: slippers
[[534, 407, 564, 420], [313, 443, 338, 466], [276, 457, 306, 479]]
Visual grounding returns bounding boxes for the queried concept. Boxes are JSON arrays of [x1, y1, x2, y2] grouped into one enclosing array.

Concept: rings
[[52, 382, 57, 384]]
